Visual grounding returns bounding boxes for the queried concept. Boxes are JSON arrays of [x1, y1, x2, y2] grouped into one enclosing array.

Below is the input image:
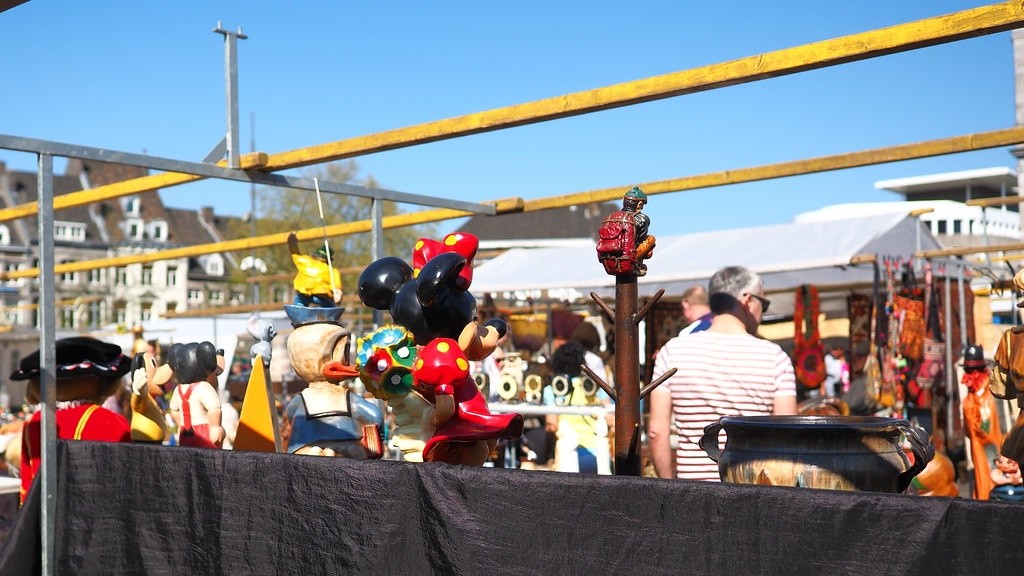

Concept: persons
[[475, 307, 614, 429], [818, 344, 851, 405], [674, 283, 715, 335], [647, 265, 799, 481]]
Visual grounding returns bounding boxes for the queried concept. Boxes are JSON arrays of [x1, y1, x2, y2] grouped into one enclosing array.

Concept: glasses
[[744, 292, 770, 314]]
